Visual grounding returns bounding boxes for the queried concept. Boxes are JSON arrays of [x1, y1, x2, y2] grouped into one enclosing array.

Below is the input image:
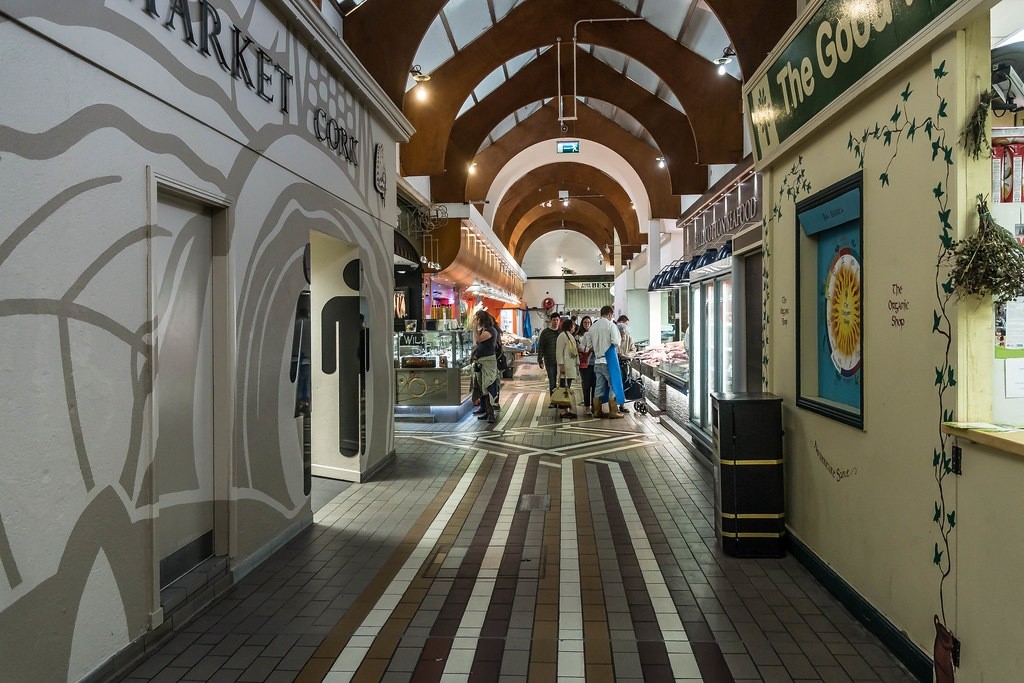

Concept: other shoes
[[560, 411, 577, 419], [586, 408, 592, 415], [548, 403, 556, 408], [493, 404, 500, 409]]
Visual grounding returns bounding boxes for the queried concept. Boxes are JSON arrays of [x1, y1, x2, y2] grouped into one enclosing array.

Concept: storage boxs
[[992, 156, 1002, 204], [1012, 155, 1023, 203]]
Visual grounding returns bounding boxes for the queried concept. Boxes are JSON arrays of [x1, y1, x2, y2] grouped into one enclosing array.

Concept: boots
[[473, 394, 486, 414], [593, 398, 609, 418], [483, 393, 495, 423], [478, 411, 488, 420], [609, 399, 624, 419]]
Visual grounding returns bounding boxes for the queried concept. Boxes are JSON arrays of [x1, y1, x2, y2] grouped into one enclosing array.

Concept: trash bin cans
[[710, 390, 790, 559]]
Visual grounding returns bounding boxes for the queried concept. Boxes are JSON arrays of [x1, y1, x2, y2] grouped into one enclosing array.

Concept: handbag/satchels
[[550, 372, 572, 406]]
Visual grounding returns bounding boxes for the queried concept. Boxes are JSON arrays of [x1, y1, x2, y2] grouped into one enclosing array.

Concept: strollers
[[618, 355, 652, 415]]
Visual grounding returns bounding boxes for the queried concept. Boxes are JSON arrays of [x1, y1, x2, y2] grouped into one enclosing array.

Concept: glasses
[[583, 321, 591, 324]]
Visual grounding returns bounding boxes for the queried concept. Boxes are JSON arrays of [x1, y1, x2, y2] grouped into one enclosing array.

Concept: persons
[[469, 310, 507, 421], [537, 306, 637, 419]]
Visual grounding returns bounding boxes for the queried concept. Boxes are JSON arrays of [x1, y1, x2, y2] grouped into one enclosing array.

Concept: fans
[[544, 298, 554, 309]]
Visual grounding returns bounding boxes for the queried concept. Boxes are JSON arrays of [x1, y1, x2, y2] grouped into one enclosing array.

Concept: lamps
[[647, 221, 759, 292], [597, 244, 641, 265], [990, 63, 1024, 113], [563, 195, 604, 206], [409, 234, 441, 270], [463, 278, 518, 305]]
